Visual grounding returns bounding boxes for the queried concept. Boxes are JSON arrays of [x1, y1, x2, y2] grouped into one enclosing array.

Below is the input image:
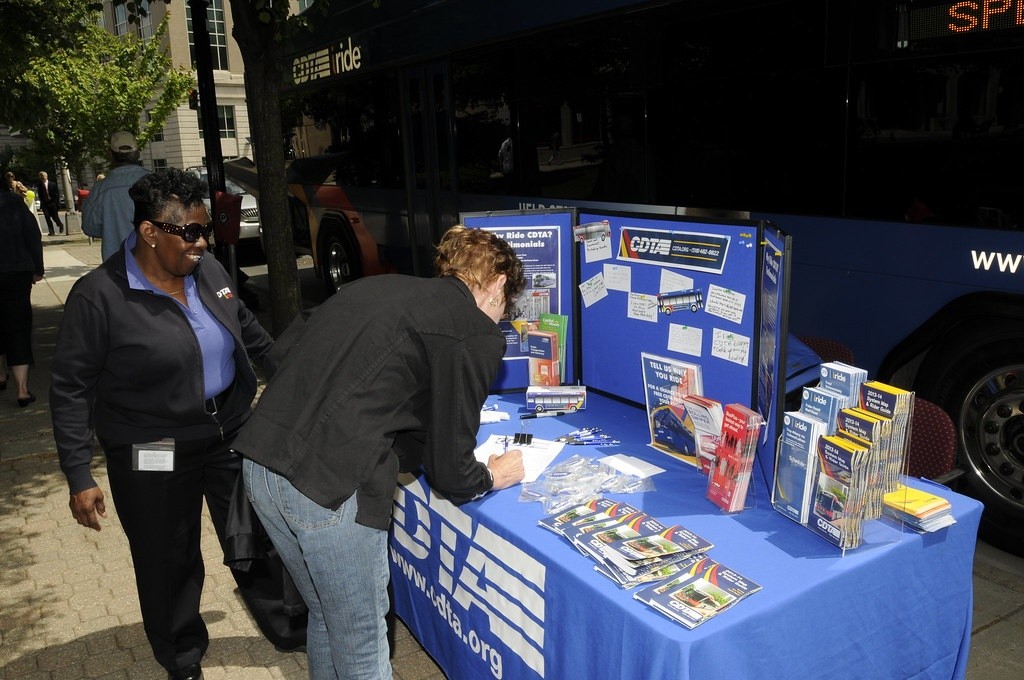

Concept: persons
[[48, 166, 310, 680], [1, 128, 161, 409], [230, 225, 529, 679]]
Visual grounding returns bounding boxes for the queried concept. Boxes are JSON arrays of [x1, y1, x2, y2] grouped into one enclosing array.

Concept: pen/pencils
[[504, 435, 509, 452], [553, 426, 620, 446]]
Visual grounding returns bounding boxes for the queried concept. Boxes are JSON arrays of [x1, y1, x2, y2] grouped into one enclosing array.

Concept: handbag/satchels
[[16, 181, 27, 198]]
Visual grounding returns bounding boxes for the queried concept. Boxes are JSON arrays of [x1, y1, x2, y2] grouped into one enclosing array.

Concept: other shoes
[[48, 231, 55, 236], [0, 371, 9, 390], [18, 392, 35, 407], [59, 223, 63, 233]]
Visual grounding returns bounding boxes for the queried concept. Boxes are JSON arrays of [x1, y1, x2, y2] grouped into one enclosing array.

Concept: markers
[[519, 410, 566, 419]]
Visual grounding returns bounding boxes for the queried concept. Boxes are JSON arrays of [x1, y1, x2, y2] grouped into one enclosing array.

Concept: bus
[[273, 0, 1024, 553]]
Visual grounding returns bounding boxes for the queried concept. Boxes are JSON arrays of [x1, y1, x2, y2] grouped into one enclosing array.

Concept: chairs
[[903, 397, 966, 494]]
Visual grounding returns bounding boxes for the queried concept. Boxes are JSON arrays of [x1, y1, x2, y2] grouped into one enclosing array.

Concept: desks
[[385, 384, 985, 680]]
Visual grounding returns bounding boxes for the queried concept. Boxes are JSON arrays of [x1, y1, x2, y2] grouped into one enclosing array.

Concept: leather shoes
[[274, 641, 307, 654], [168, 663, 201, 680]]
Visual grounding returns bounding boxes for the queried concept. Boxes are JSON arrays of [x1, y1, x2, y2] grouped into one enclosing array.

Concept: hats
[[110, 131, 138, 153]]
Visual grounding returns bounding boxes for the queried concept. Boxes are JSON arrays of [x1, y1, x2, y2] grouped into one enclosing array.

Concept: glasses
[[137, 218, 214, 242], [8, 176, 13, 177]]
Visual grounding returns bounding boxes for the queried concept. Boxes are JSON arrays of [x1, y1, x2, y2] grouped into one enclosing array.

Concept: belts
[[205, 386, 233, 416]]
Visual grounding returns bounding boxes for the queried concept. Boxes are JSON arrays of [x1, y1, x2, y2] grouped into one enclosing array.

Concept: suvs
[[185, 157, 266, 263]]
